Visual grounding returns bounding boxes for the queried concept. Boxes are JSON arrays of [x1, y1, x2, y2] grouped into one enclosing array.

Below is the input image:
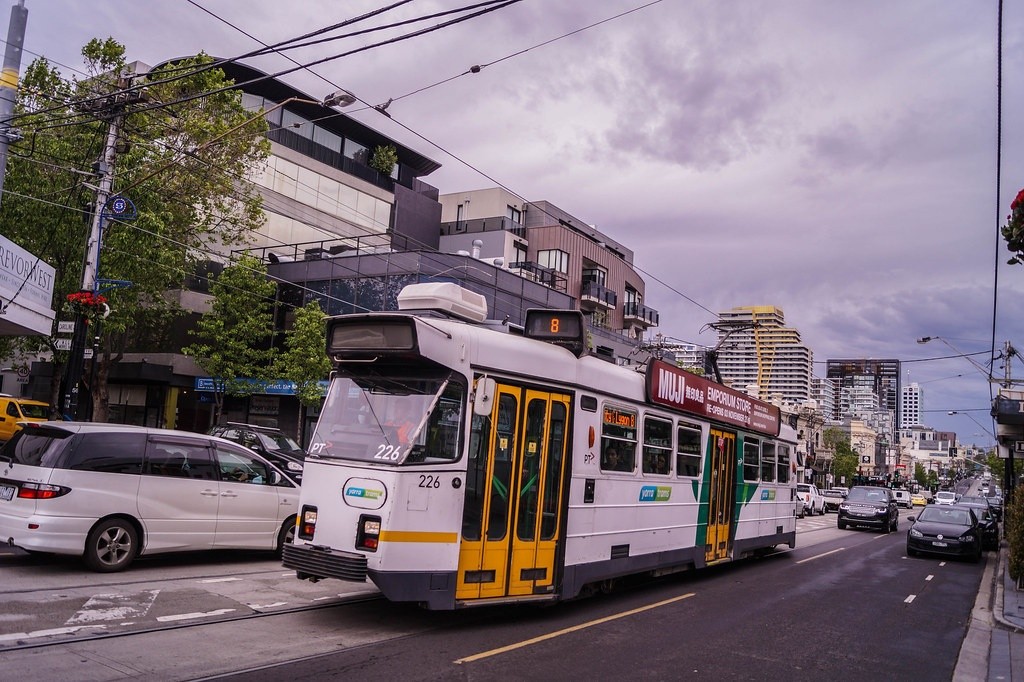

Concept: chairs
[[957, 513, 969, 523], [929, 510, 943, 522], [149, 449, 212, 479]]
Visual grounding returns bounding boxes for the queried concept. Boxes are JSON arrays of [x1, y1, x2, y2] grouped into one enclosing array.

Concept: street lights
[[805, 407, 838, 483], [888, 441, 914, 487], [859, 432, 892, 485], [918, 334, 1014, 505], [63, 91, 352, 415], [948, 412, 1010, 539]]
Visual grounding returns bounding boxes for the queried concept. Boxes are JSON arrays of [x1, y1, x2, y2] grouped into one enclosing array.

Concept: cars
[[906, 503, 986, 562], [796, 495, 806, 518], [911, 494, 927, 507], [983, 487, 990, 495], [982, 481, 989, 486], [956, 493, 964, 501], [953, 502, 1000, 551], [955, 495, 995, 523], [986, 496, 1003, 521], [821, 490, 846, 510], [832, 487, 850, 497]]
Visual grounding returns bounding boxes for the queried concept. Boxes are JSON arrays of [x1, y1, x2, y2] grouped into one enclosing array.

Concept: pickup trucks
[[797, 484, 827, 516]]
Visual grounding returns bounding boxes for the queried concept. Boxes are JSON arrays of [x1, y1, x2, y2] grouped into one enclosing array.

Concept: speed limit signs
[[18, 365, 31, 377]]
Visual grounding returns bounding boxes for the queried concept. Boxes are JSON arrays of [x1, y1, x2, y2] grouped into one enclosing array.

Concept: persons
[[387, 399, 420, 445]]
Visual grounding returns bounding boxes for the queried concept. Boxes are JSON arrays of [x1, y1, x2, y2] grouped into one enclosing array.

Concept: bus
[[279, 281, 800, 614]]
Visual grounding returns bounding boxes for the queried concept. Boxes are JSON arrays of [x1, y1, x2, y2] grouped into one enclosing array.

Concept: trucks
[[983, 472, 991, 481]]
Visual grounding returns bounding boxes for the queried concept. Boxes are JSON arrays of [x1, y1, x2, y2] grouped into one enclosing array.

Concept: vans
[[0, 394, 63, 443], [933, 492, 957, 510]]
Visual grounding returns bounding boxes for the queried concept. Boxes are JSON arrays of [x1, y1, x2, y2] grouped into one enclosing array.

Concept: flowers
[[62, 292, 108, 326]]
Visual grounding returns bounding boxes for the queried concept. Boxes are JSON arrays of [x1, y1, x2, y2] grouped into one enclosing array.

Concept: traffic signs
[[54, 338, 72, 350]]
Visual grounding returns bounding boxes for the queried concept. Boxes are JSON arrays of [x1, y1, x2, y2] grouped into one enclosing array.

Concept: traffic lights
[[894, 471, 899, 478]]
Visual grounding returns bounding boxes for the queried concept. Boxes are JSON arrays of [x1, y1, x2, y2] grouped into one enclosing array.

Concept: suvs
[[836, 485, 900, 534], [0, 418, 301, 572], [939, 485, 952, 492], [206, 419, 307, 486], [919, 491, 935, 504], [892, 490, 913, 509]]
